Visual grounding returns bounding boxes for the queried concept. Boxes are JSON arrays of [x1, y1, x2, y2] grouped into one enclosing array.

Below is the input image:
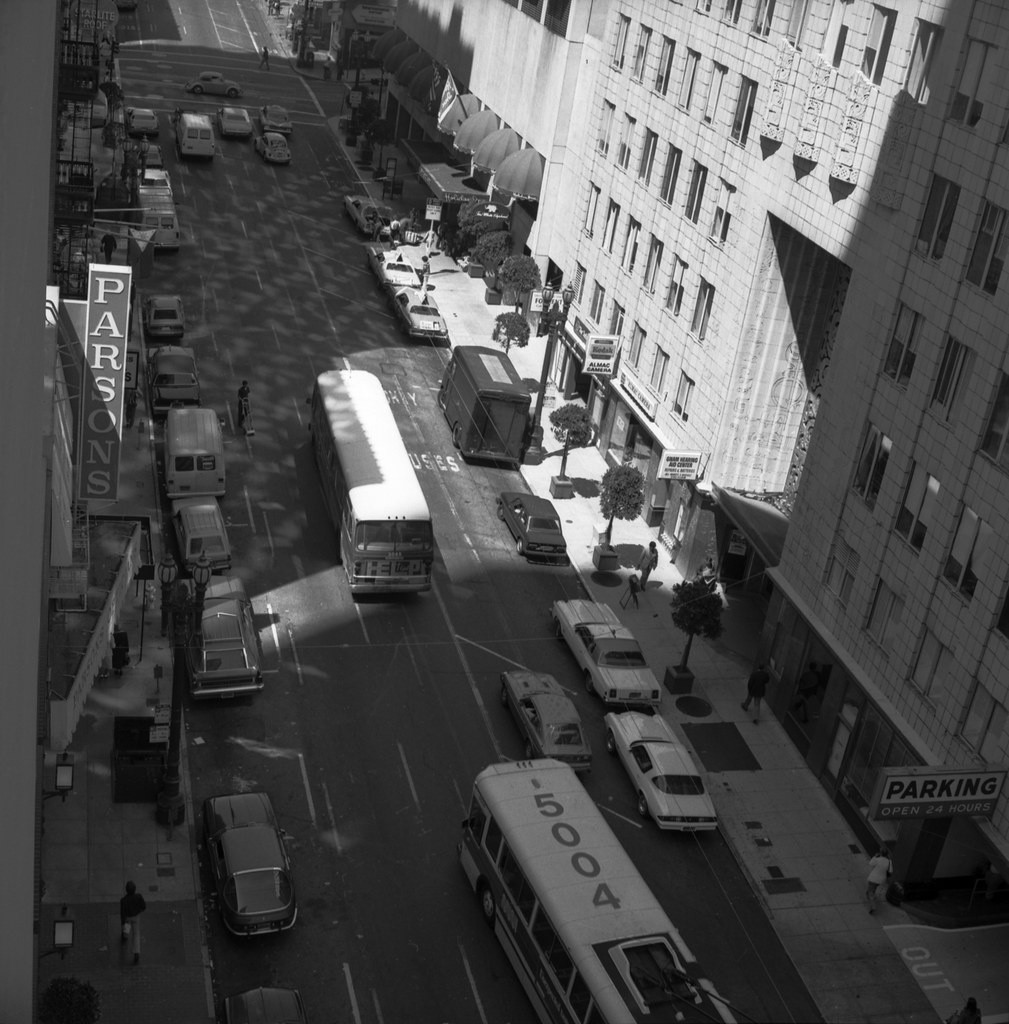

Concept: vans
[[163, 409, 226, 498], [176, 111, 217, 158]]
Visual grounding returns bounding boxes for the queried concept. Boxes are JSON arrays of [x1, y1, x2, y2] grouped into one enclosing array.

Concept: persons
[[145, 583, 156, 609], [959, 997, 981, 1024], [636, 541, 658, 591], [526, 708, 537, 720], [267, 0, 280, 15], [101, 230, 117, 264], [259, 46, 270, 71], [337, 62, 343, 81], [419, 256, 430, 289], [125, 388, 143, 428], [323, 57, 332, 80], [370, 211, 401, 242], [99, 624, 129, 679], [793, 660, 822, 722], [741, 664, 769, 725], [119, 881, 146, 966], [866, 846, 893, 913], [104, 72, 110, 83], [238, 380, 250, 429], [695, 556, 716, 593]]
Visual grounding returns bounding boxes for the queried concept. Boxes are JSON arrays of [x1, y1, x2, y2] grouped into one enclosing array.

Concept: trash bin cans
[[112, 631, 130, 669]]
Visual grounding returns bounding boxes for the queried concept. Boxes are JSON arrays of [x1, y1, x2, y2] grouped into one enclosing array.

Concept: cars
[[130, 108, 181, 249], [368, 246, 421, 289], [220, 108, 254, 137], [187, 71, 242, 99], [499, 670, 593, 770], [495, 490, 569, 560], [551, 599, 662, 706], [177, 576, 266, 699], [603, 710, 717, 831], [149, 345, 201, 413], [171, 496, 233, 572], [202, 792, 298, 937], [258, 105, 294, 131], [254, 133, 291, 162], [145, 293, 185, 338], [345, 194, 396, 237], [390, 287, 449, 340]]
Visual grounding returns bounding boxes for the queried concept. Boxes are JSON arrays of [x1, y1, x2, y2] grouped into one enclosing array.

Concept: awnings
[[370, 31, 546, 202]]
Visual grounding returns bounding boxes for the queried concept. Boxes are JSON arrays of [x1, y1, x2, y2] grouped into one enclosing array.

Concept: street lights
[[122, 136, 132, 175], [140, 135, 151, 177], [524, 281, 576, 464]]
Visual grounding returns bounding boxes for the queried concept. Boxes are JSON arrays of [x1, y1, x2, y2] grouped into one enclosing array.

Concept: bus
[[437, 345, 534, 464], [458, 759, 741, 1024], [308, 369, 438, 594]]
